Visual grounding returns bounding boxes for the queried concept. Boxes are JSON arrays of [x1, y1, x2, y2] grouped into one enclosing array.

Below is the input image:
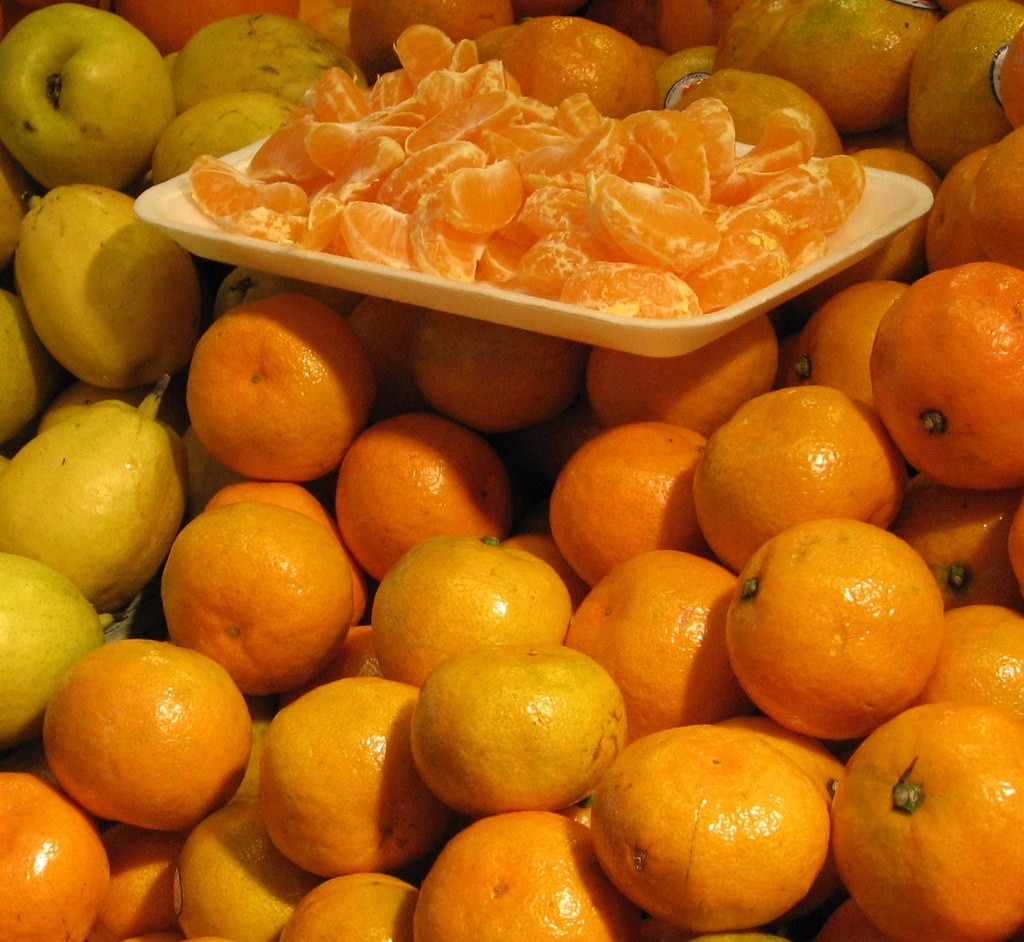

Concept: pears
[[0, 0, 367, 753]]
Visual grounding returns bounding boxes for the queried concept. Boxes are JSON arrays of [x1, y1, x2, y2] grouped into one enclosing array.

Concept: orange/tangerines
[[117, 0, 1024, 612], [0, 502, 1024, 942]]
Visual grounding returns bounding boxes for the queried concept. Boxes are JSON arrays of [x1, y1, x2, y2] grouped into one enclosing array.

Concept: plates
[[132, 134, 936, 358]]
